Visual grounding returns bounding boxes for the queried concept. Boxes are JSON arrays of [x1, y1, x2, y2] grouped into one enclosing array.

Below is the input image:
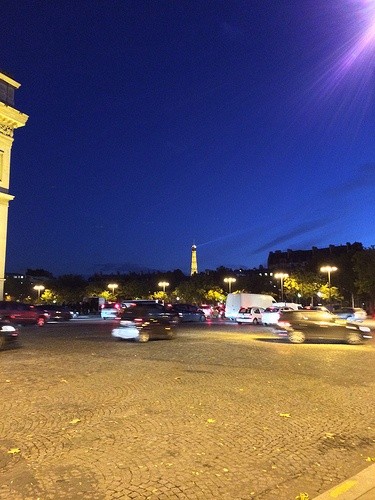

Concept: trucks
[[225, 293, 277, 319]]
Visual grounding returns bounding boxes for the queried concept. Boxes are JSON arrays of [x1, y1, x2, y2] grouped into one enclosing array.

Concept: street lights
[[320, 266, 337, 305], [274, 273, 289, 302], [224, 278, 236, 293], [158, 282, 169, 292], [34, 285, 45, 302], [108, 284, 118, 295]]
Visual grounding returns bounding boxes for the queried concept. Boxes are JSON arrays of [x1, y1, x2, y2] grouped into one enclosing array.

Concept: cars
[[101, 303, 225, 343], [236, 306, 294, 326], [0, 301, 79, 349]]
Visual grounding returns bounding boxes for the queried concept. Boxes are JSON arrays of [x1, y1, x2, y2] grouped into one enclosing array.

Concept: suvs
[[278, 307, 373, 345]]
